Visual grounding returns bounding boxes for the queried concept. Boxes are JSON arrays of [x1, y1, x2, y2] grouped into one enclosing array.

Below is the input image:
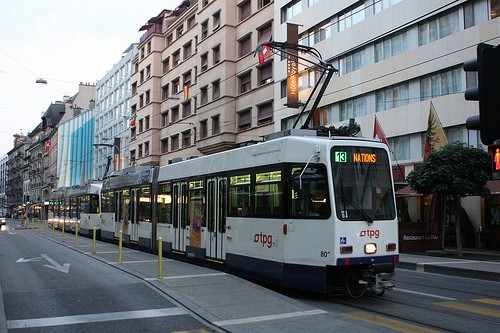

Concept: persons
[[317, 190, 344, 215]]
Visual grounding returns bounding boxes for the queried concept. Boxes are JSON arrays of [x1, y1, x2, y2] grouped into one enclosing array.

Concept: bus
[[46, 40, 400, 299]]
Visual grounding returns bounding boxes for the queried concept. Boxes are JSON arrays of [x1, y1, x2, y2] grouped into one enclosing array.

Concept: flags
[[114, 137, 121, 171], [286, 23, 300, 107], [373, 117, 390, 154], [422, 100, 450, 163]]
